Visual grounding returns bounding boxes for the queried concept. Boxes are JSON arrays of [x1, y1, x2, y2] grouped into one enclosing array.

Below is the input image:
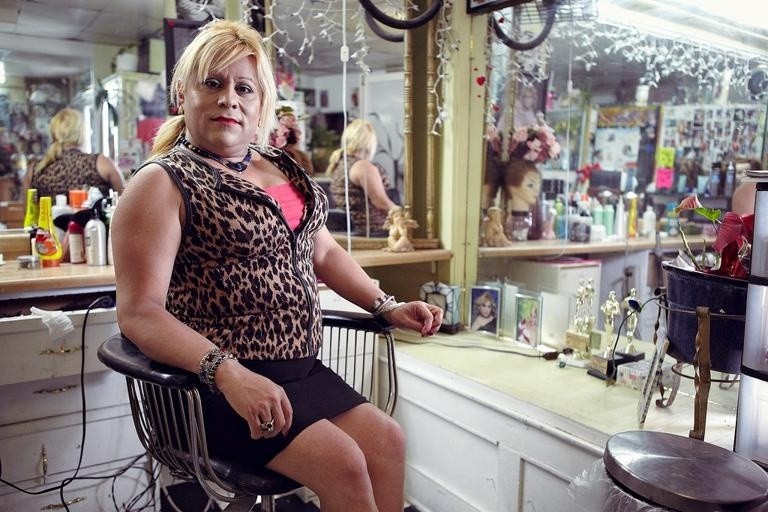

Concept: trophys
[[584, 279, 604, 352], [615, 288, 645, 360], [587, 287, 626, 381], [565, 277, 589, 353]]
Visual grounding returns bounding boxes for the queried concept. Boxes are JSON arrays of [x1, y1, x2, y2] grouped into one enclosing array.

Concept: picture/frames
[[468, 284, 503, 336], [513, 295, 543, 350]]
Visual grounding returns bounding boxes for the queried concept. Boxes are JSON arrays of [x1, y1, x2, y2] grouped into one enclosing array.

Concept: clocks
[[418, 280, 466, 336]]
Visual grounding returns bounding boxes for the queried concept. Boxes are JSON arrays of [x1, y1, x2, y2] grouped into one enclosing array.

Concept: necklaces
[[178, 135, 252, 172]]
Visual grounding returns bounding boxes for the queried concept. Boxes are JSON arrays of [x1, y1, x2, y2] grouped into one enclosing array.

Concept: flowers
[[507, 118, 561, 166], [483, 112, 503, 160]]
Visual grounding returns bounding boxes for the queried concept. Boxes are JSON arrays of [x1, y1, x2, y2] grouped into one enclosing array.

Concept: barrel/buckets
[[661, 260, 748, 375]]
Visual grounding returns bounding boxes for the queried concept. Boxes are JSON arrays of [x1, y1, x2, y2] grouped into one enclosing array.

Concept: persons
[[503, 161, 542, 212], [471, 290, 497, 335], [517, 303, 537, 347], [109, 15, 444, 512], [22, 109, 125, 214], [324, 119, 403, 237]]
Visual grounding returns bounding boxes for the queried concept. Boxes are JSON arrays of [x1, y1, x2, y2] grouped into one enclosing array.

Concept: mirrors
[[0, 1, 179, 235], [474, 2, 767, 411], [264, 0, 415, 244], [510, 1, 767, 229], [487, 1, 516, 159]]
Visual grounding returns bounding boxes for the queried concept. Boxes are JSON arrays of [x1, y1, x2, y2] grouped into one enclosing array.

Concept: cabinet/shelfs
[[1, 285, 165, 512], [314, 276, 378, 406], [510, 258, 602, 336]]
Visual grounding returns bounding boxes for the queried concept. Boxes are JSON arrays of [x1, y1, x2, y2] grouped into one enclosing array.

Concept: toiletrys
[[23, 189, 38, 229], [528, 192, 657, 243], [35, 188, 115, 267]]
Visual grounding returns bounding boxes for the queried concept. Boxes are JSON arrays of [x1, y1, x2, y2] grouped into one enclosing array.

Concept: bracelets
[[196, 347, 236, 388], [371, 289, 408, 317]]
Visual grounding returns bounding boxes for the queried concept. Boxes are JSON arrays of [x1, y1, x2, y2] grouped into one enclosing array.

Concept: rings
[[260, 418, 276, 435]]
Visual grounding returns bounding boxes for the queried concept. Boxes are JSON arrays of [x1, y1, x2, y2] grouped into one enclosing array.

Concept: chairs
[[97, 311, 397, 510], [324, 206, 386, 238]]
